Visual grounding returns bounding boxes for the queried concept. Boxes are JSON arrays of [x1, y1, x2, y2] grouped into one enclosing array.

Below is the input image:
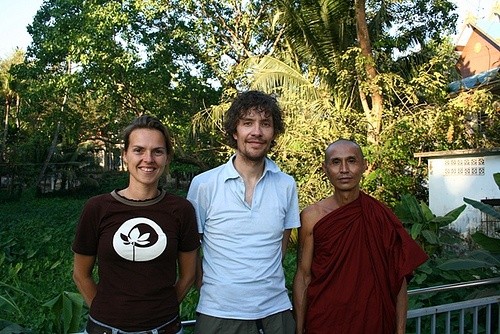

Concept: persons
[[71, 115, 200, 334], [186, 90, 302, 334], [293, 139, 429, 334]]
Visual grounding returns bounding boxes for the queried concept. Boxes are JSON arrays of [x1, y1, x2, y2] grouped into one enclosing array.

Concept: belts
[[85, 315, 182, 334]]
